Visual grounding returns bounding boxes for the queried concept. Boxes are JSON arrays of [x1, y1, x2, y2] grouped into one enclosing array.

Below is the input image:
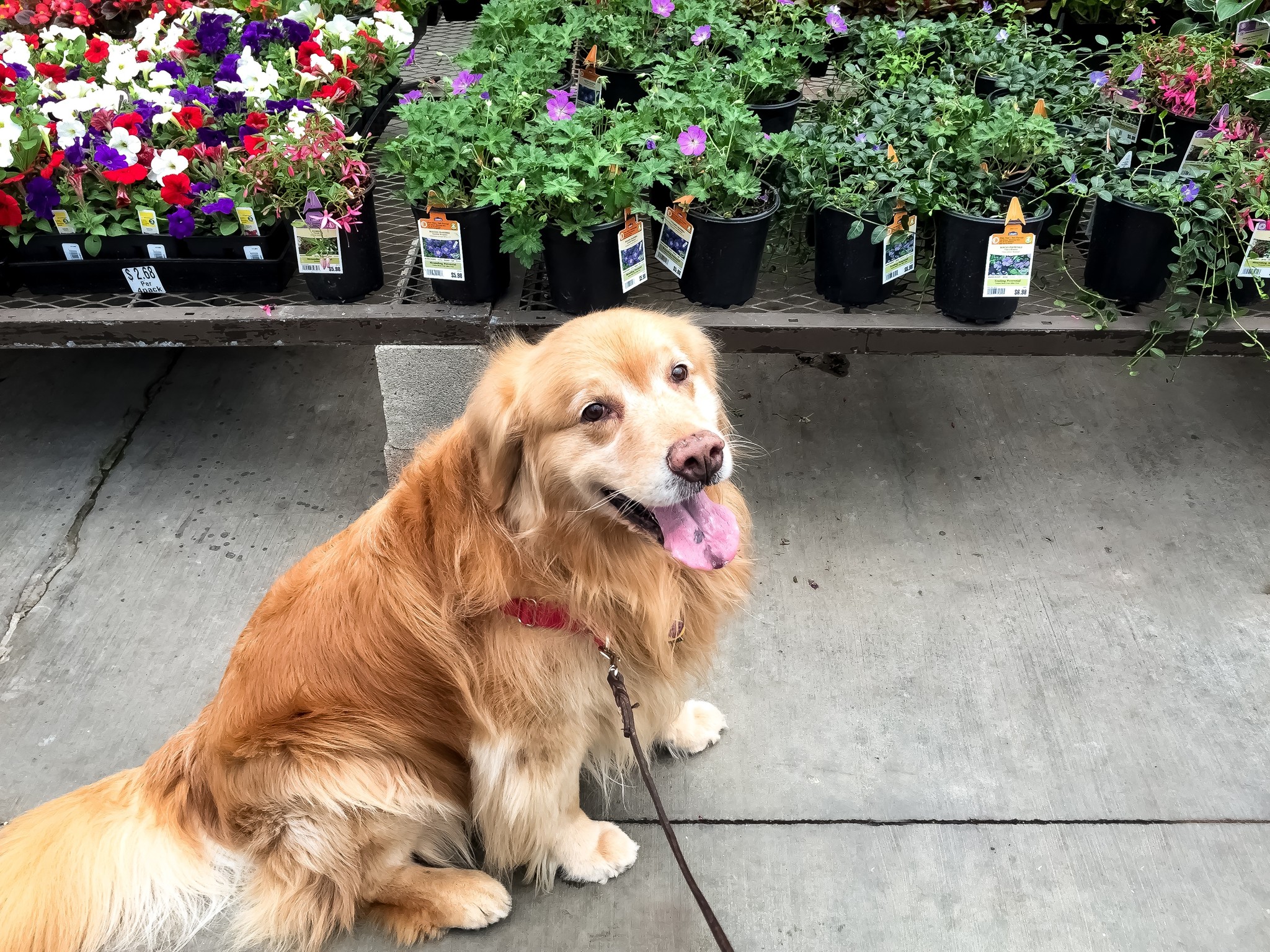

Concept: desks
[[577, 48, 655, 113]]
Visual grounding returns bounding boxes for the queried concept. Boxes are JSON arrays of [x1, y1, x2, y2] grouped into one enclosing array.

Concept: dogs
[[3, 299, 773, 952]]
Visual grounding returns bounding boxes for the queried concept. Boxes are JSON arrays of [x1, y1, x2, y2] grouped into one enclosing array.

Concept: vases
[[670, 176, 780, 309], [538, 217, 623, 315], [427, 2, 443, 26], [400, 15, 427, 53], [343, 74, 403, 158], [437, 0, 490, 21], [1085, 167, 1187, 305], [0, 0, 1270, 352], [1146, 97, 1211, 171], [291, 172, 385, 304], [411, 198, 510, 306], [934, 188, 1052, 325], [746, 88, 803, 134], [809, 178, 895, 310], [648, 173, 685, 252]]
